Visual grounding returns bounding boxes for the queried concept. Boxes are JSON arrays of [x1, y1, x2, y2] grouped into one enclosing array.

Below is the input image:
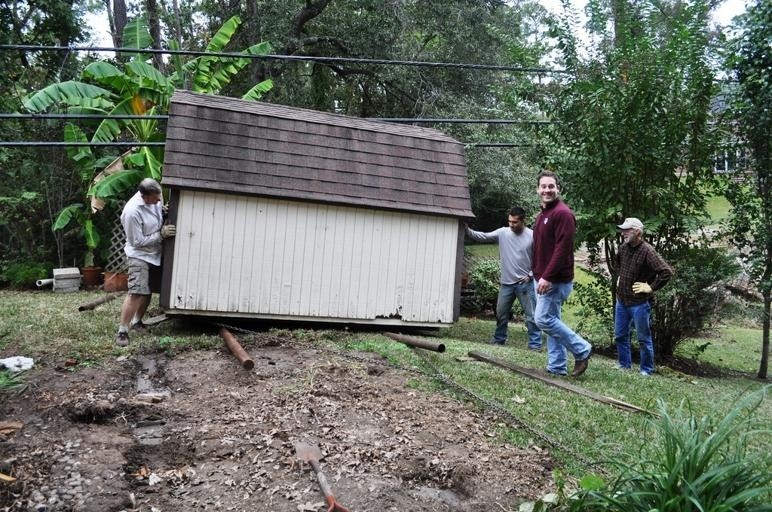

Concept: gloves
[[162, 201, 170, 216], [161, 219, 176, 239]]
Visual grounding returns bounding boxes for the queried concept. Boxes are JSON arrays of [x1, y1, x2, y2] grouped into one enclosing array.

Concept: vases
[[81, 267, 102, 285], [104, 271, 127, 292]]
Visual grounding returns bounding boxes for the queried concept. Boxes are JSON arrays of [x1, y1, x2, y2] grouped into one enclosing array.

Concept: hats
[[616, 217, 644, 234]]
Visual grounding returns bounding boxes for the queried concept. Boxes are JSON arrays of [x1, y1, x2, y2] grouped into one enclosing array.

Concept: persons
[[610, 217, 673, 378], [114, 176, 176, 347], [464, 207, 541, 352], [526, 171, 596, 378]]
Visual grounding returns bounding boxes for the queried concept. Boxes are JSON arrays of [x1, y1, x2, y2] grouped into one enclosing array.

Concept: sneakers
[[571, 348, 593, 375], [129, 320, 147, 330], [116, 331, 130, 347]]
